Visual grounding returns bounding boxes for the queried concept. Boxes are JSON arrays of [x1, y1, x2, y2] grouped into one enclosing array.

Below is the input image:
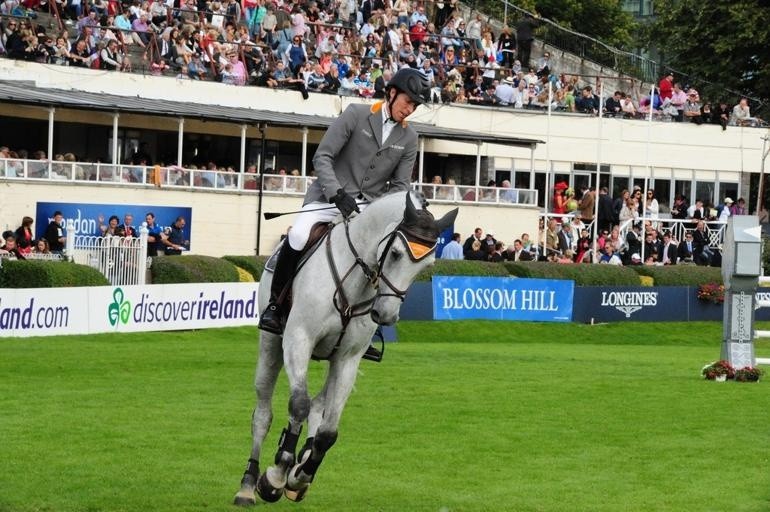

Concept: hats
[[724, 198, 734, 203], [665, 72, 675, 76]]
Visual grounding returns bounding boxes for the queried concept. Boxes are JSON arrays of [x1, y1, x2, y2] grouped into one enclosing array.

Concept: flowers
[[695, 283, 725, 305], [703, 360, 766, 383]]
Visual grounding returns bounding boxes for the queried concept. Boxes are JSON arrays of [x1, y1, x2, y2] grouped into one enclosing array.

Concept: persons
[[257, 67, 437, 363]]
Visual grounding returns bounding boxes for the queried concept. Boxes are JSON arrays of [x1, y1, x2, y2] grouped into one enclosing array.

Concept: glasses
[[637, 191, 652, 198], [291, 34, 455, 91]]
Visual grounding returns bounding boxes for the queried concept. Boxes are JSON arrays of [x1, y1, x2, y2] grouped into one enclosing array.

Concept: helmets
[[386, 66, 432, 108]]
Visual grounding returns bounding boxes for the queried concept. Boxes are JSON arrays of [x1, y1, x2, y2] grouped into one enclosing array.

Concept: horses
[[235, 191, 458, 507]]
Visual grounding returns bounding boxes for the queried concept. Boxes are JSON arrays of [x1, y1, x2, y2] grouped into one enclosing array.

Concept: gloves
[[331, 189, 360, 218]]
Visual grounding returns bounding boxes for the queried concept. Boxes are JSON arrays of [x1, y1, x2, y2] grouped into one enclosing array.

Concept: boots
[[363, 346, 382, 361], [261, 234, 302, 330]]
